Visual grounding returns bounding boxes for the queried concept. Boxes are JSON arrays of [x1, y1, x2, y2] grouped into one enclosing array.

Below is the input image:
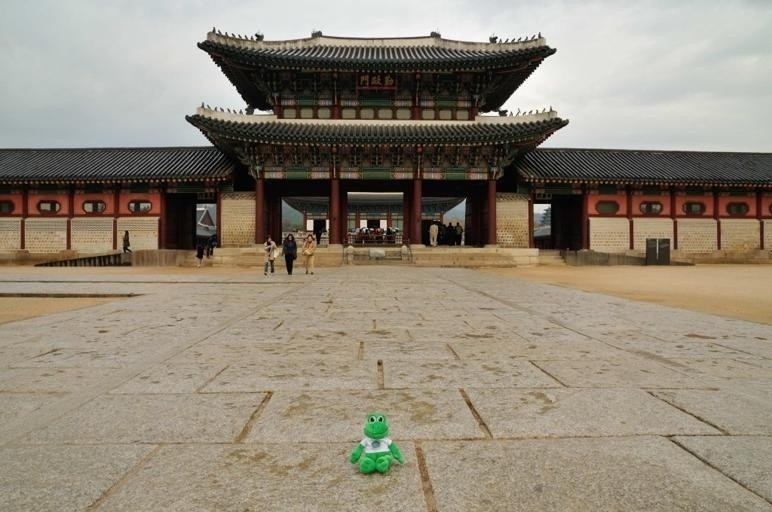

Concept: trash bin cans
[[646, 238, 670, 264]]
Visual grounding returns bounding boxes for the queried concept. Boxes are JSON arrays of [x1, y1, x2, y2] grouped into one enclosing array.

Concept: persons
[[262, 235, 278, 275], [351, 227, 399, 244], [422, 219, 463, 246], [313, 223, 323, 244], [122, 229, 131, 253], [302, 234, 317, 275], [206, 234, 215, 257], [195, 241, 206, 267], [282, 233, 297, 276]]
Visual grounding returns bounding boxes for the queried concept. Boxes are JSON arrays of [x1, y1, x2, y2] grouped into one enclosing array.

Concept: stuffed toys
[[351, 412, 405, 476]]
